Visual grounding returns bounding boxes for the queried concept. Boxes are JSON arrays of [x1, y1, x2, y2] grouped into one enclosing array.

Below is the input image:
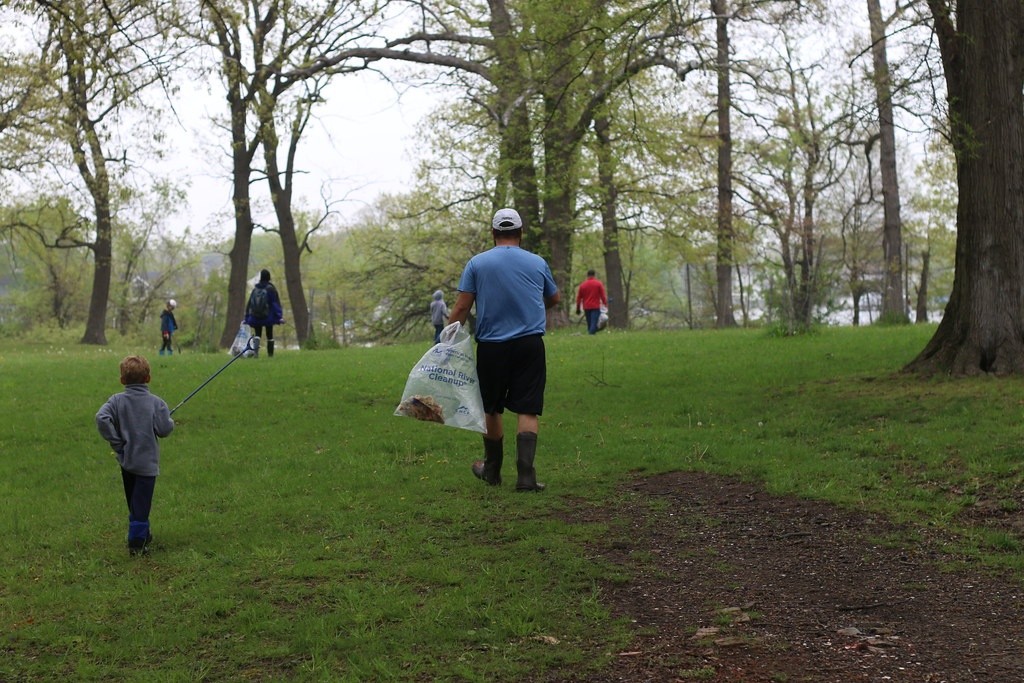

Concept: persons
[[96, 355, 174, 554], [244, 269, 285, 358], [448, 207, 560, 491], [160, 299, 177, 355], [575, 270, 608, 334], [430, 290, 449, 345]]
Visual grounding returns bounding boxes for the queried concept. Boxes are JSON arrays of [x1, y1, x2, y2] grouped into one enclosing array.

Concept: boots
[[128, 515, 153, 545], [253, 336, 260, 358], [129, 521, 151, 563], [267, 339, 274, 357], [168, 350, 172, 356], [515, 431, 545, 491], [472, 434, 505, 486], [160, 349, 164, 356]]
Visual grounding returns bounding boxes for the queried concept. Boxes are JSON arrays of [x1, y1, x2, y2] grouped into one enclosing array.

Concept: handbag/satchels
[[229, 320, 255, 359]]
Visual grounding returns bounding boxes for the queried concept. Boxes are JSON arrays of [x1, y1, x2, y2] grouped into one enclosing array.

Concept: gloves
[[576, 310, 581, 315]]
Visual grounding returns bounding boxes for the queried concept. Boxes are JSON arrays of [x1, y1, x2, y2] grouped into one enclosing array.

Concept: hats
[[167, 299, 176, 309], [492, 208, 522, 231]]
